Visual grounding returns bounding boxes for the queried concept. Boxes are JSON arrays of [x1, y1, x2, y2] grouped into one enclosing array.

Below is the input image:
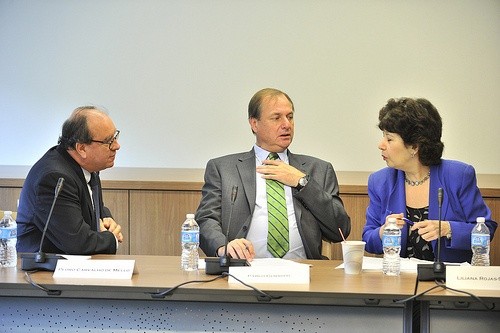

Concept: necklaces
[[404, 169, 430, 186]]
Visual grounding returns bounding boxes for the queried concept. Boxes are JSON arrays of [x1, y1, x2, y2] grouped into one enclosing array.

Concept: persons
[[192, 89, 351, 264], [16, 106, 124, 255], [361, 98, 498, 266]]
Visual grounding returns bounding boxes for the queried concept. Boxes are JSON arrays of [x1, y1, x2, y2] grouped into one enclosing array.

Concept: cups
[[341, 241, 366, 275]]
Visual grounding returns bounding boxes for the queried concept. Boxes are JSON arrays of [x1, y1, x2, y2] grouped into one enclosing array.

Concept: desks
[[0, 234, 500, 333]]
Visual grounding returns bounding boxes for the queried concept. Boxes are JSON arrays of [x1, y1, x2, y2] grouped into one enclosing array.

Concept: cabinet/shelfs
[[0, 149, 500, 255]]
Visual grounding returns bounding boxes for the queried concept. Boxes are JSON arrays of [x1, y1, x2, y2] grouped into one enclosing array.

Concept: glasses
[[91, 130, 120, 150]]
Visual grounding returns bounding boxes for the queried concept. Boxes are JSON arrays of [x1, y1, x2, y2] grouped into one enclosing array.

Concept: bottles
[[382, 217, 402, 276], [471, 217, 491, 266], [0, 210, 18, 267], [181, 214, 201, 270]]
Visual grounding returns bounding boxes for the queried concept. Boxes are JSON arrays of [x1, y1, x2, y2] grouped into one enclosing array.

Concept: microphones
[[205, 185, 251, 276], [20, 177, 68, 272], [418, 188, 447, 283]]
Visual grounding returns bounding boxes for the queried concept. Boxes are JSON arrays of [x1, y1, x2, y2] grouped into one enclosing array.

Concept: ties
[[89, 173, 101, 232], [266, 152, 290, 259]]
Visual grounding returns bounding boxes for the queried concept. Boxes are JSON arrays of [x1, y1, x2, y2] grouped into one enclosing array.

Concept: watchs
[[295, 175, 309, 192]]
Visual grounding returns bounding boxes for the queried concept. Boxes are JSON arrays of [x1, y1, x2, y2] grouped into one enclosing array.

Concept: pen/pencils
[[401, 217, 414, 226]]
